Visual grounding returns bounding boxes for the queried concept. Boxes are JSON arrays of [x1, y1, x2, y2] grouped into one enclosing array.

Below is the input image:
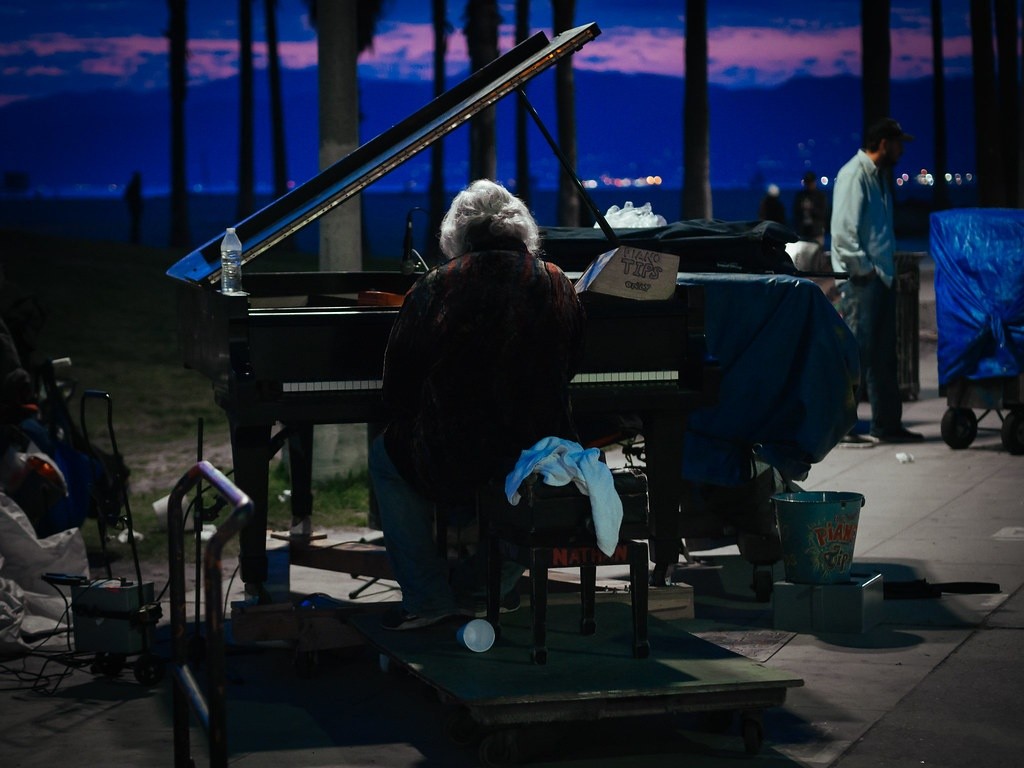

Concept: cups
[[456, 619, 496, 652]]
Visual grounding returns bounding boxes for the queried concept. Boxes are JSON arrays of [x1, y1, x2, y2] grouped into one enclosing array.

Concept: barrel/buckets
[[770, 491, 866, 585]]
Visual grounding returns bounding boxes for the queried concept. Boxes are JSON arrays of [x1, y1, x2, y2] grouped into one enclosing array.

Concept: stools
[[479, 464, 649, 665]]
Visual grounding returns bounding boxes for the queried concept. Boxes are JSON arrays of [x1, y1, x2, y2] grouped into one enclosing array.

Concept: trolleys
[[40, 390, 168, 689], [925, 208, 1024, 450]]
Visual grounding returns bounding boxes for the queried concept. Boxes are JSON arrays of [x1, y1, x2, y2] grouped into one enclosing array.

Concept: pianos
[[164, 22, 709, 586]]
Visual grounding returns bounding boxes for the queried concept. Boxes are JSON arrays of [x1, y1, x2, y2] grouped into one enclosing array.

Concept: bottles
[[221, 228, 243, 293]]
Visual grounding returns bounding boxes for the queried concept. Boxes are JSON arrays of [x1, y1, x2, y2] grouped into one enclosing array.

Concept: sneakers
[[841, 432, 860, 444], [870, 429, 925, 441], [379, 604, 460, 630], [457, 590, 522, 617]]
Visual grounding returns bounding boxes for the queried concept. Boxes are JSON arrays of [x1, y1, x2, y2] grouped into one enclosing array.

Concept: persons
[[830, 117, 928, 448], [758, 187, 788, 226], [122, 169, 149, 243], [791, 173, 829, 246], [368, 178, 586, 637]]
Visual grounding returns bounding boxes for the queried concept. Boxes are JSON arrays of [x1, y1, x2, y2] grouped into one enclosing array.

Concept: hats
[[865, 117, 915, 142]]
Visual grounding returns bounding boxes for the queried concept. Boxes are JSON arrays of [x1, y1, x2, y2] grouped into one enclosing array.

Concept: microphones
[[399, 210, 415, 277]]
